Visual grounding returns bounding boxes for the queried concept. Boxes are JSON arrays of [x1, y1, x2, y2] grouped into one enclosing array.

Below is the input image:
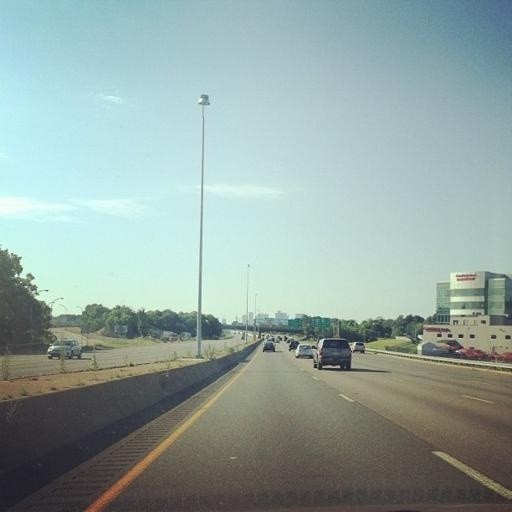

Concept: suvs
[[348, 342, 365, 354], [314, 338, 352, 370], [47, 337, 82, 359]]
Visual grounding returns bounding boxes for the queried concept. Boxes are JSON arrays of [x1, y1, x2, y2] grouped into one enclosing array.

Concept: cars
[[295, 343, 314, 359], [233, 331, 264, 338], [263, 334, 299, 352]]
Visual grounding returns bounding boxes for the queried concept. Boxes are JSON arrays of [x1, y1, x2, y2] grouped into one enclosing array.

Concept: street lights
[[29, 290, 83, 338], [254, 294, 258, 322], [197, 94, 210, 358], [246, 264, 250, 334]]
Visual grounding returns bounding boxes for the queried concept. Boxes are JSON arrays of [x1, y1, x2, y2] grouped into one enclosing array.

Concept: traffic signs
[[288, 319, 303, 327], [311, 317, 331, 327]]
